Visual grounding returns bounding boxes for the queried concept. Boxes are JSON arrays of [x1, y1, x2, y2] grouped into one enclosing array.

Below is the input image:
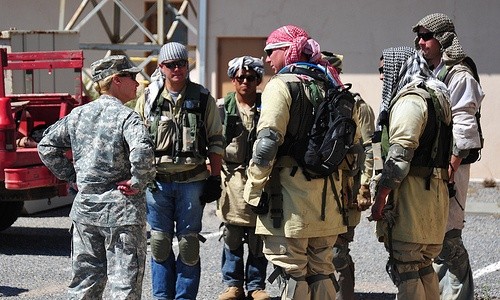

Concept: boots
[[334, 275, 355, 299]]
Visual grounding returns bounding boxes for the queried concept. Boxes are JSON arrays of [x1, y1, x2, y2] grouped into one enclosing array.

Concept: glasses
[[417, 31, 446, 41], [378, 65, 384, 73], [265, 48, 272, 56], [161, 58, 187, 68], [233, 74, 257, 82], [117, 72, 137, 80]]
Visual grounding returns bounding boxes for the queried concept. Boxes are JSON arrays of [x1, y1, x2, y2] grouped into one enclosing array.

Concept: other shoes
[[251, 288, 271, 299], [218, 285, 244, 299]]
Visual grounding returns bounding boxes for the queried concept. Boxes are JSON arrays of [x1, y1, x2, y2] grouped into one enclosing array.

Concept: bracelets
[[449, 162, 455, 171]]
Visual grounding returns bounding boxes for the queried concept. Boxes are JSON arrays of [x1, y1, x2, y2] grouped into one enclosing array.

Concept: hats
[[91, 54, 143, 83], [227, 55, 265, 77]]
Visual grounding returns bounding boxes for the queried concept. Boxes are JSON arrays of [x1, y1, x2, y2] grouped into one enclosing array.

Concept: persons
[[133, 13, 484, 300], [36, 54, 153, 300]]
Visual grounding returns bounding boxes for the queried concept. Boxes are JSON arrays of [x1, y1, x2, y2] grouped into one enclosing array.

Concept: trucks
[[0, 40, 164, 230]]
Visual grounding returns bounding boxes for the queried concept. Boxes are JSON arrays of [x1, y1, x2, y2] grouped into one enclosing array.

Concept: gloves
[[352, 184, 371, 211], [249, 191, 270, 214], [203, 174, 222, 202]]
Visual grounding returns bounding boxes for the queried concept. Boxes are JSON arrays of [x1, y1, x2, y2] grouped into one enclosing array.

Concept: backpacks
[[272, 63, 357, 181]]
